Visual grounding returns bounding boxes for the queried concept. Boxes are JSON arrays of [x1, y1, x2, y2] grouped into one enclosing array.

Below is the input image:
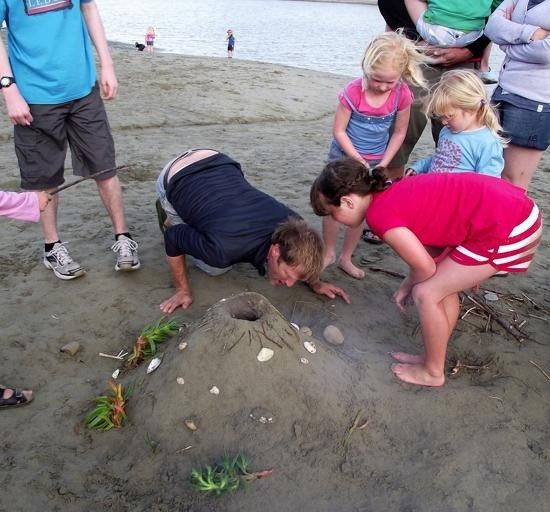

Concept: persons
[[310, 155, 533, 387], [227, 30, 235, 58], [0, 1, 142, 278], [145, 27, 155, 53], [384, 0, 499, 84], [317, 27, 437, 280], [0, 190, 53, 408], [135, 43, 145, 51], [404, 70, 511, 179], [483, 0, 550, 191], [154, 149, 350, 314], [361, 0, 492, 245]]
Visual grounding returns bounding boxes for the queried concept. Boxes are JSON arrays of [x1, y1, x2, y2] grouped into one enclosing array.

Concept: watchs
[[0, 76, 16, 90]]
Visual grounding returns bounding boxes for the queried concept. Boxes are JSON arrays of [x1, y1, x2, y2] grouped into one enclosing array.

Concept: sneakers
[[114, 235, 141, 272], [44, 242, 86, 280]]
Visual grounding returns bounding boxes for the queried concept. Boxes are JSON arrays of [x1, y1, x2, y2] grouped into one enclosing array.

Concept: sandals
[[362, 223, 383, 244], [0, 388, 33, 409]]
